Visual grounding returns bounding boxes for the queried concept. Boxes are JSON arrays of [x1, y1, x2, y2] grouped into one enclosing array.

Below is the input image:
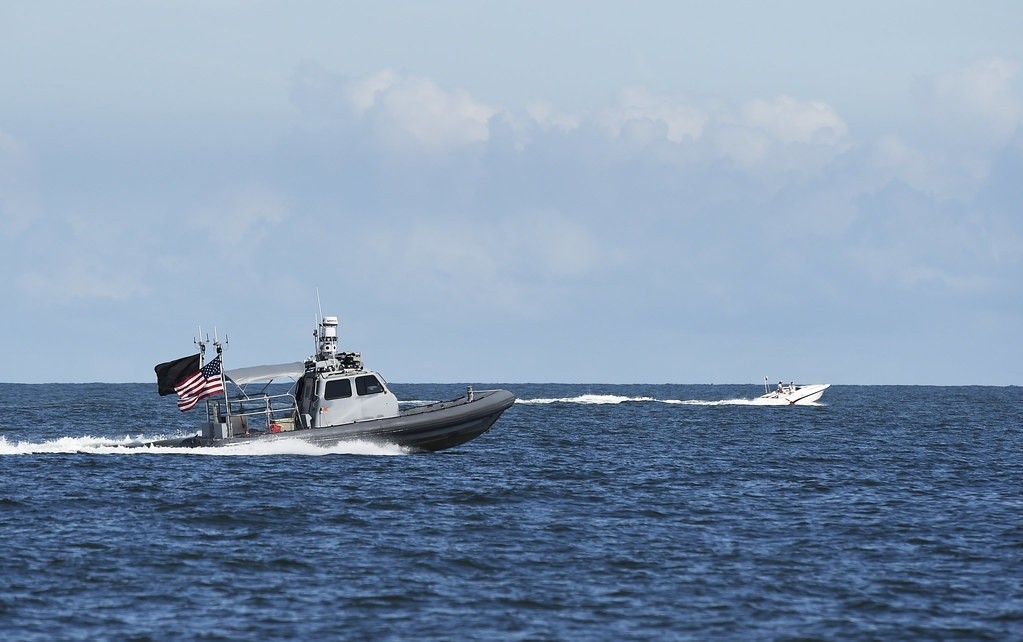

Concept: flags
[[174, 354, 225, 413], [155, 354, 200, 396]]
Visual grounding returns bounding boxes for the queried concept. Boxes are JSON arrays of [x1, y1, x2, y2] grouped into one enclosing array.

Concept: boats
[[155, 287, 516, 452], [755, 377, 831, 405]]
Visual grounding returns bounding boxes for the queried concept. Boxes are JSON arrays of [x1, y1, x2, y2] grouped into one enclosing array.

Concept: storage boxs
[[270, 418, 295, 432]]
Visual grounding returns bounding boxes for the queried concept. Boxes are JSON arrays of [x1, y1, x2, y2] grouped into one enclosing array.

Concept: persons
[[789, 382, 796, 394], [778, 381, 783, 392]]
